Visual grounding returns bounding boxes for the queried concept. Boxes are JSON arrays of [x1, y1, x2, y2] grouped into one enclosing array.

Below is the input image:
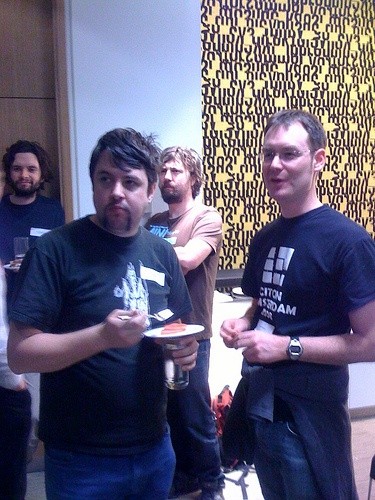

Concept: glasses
[[256, 149, 312, 163]]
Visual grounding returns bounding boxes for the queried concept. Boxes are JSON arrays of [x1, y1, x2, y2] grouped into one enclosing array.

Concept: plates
[[142, 323, 206, 337], [3, 263, 22, 270], [232, 287, 254, 300]]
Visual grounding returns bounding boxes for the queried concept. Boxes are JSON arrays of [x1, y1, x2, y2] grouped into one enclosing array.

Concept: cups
[[13, 237, 29, 260], [163, 342, 191, 391]]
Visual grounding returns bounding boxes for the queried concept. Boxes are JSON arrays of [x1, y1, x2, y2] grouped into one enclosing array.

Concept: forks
[[116, 308, 192, 325]]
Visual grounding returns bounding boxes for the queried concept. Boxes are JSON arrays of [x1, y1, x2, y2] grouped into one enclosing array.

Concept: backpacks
[[211, 385, 253, 499]]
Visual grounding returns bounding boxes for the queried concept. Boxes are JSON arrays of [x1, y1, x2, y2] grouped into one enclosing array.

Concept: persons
[[143, 145, 226, 500], [219, 108, 375, 500], [0, 140, 66, 500], [7, 128, 199, 500]]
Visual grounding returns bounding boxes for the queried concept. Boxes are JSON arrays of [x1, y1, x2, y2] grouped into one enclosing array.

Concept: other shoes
[[25, 455, 46, 472], [26, 444, 38, 462], [200, 488, 215, 500], [168, 484, 199, 498]]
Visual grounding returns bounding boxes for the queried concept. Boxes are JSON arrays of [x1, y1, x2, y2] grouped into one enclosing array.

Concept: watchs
[[288, 334, 303, 363]]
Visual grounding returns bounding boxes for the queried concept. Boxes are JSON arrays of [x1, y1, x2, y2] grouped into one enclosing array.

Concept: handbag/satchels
[[220, 377, 256, 468]]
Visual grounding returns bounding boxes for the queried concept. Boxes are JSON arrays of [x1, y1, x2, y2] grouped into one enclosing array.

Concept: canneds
[[163, 338, 189, 390]]
